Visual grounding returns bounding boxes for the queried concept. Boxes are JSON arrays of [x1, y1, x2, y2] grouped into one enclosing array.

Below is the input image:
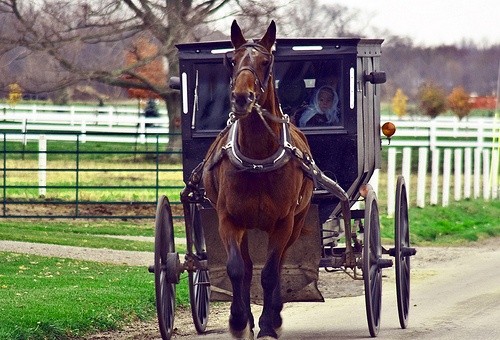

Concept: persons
[[294, 85, 344, 127]]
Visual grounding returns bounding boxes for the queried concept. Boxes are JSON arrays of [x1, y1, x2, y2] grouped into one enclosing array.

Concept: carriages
[[147, 18, 416, 340]]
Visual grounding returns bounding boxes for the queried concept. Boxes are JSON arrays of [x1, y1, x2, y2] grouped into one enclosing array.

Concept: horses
[[202, 19, 316, 340]]
